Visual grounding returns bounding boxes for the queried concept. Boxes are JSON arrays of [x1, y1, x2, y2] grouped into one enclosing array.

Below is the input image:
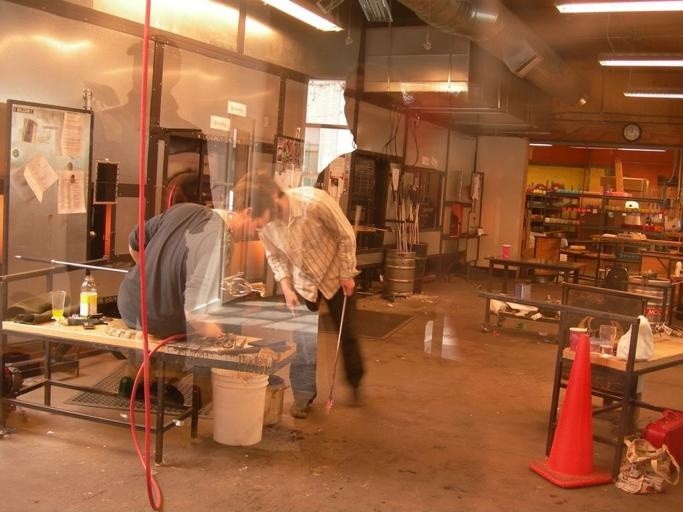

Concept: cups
[[501, 245, 510, 260], [569, 327, 588, 351], [600, 325, 616, 356]]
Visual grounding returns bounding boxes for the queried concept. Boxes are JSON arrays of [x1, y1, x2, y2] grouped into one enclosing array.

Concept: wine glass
[[50, 291, 65, 329]]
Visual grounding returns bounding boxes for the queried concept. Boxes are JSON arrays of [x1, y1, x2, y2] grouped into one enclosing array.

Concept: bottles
[[79, 276, 98, 319], [675, 261, 682, 277]]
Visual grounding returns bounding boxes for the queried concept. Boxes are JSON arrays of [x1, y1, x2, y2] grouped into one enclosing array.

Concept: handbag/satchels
[[615, 439, 671, 494]]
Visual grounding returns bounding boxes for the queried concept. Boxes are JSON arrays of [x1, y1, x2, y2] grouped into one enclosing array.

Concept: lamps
[[260, 0, 345, 32], [552, 0, 683, 19], [597, 54, 683, 100]]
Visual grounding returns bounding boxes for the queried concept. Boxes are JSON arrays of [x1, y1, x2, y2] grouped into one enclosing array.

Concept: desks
[[2, 314, 297, 466], [545, 281, 683, 479], [483, 255, 587, 291]]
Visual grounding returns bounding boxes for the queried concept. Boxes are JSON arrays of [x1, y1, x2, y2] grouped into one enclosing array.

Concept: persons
[[238, 171, 364, 419], [117, 183, 278, 406]]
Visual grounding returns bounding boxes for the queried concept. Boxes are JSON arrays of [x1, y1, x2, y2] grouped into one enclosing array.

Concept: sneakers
[[118, 376, 184, 405], [290, 391, 313, 418]]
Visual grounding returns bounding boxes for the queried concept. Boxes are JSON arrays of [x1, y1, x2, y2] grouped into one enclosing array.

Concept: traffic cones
[[530, 335, 614, 488]]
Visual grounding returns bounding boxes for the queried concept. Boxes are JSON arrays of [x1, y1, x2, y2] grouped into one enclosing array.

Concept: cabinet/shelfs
[[159, 128, 278, 285], [525, 180, 683, 240], [350, 149, 485, 268]]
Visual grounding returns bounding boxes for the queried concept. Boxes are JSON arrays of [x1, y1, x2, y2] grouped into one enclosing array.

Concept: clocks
[[622, 121, 641, 142]]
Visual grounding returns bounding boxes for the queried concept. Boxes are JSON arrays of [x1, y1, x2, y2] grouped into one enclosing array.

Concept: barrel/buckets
[[384, 249, 415, 297], [395, 241, 428, 294], [627, 276, 671, 331], [264, 376, 290, 426]]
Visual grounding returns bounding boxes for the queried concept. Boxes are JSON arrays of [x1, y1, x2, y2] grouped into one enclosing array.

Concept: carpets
[[64, 357, 214, 417], [321, 308, 416, 341]]
